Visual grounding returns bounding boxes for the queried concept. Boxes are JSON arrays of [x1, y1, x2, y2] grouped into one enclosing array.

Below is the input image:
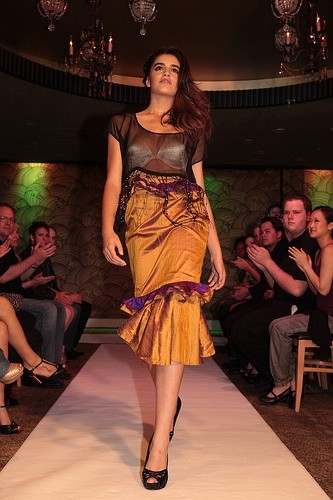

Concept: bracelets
[[31, 265, 40, 270]]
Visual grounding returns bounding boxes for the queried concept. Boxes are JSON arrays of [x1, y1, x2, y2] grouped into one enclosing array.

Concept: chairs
[[291, 336, 333, 412]]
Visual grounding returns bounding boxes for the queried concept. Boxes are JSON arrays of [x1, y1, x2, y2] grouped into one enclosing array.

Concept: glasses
[[0, 216, 15, 224]]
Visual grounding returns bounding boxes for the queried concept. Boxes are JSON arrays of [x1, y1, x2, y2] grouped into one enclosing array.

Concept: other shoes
[[67, 350, 84, 360]]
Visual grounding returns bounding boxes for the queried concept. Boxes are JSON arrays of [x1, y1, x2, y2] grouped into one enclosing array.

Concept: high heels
[[22, 358, 66, 384], [239, 361, 267, 383], [142, 433, 169, 490], [259, 385, 296, 408], [0, 363, 24, 384], [0, 406, 23, 435], [169, 396, 181, 442]]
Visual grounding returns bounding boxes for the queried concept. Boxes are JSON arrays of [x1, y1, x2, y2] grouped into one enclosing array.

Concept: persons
[[215, 193, 333, 405], [0, 201, 94, 434], [100, 45, 227, 490]]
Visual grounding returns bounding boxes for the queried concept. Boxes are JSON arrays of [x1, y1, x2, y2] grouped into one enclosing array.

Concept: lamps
[[37, 0, 68, 32], [63, 0, 120, 97], [271, 0, 333, 87], [128, 0, 159, 36]]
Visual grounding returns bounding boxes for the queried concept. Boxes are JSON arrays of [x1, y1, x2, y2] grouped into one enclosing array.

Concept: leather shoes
[[4, 383, 19, 409], [24, 371, 71, 388]]
